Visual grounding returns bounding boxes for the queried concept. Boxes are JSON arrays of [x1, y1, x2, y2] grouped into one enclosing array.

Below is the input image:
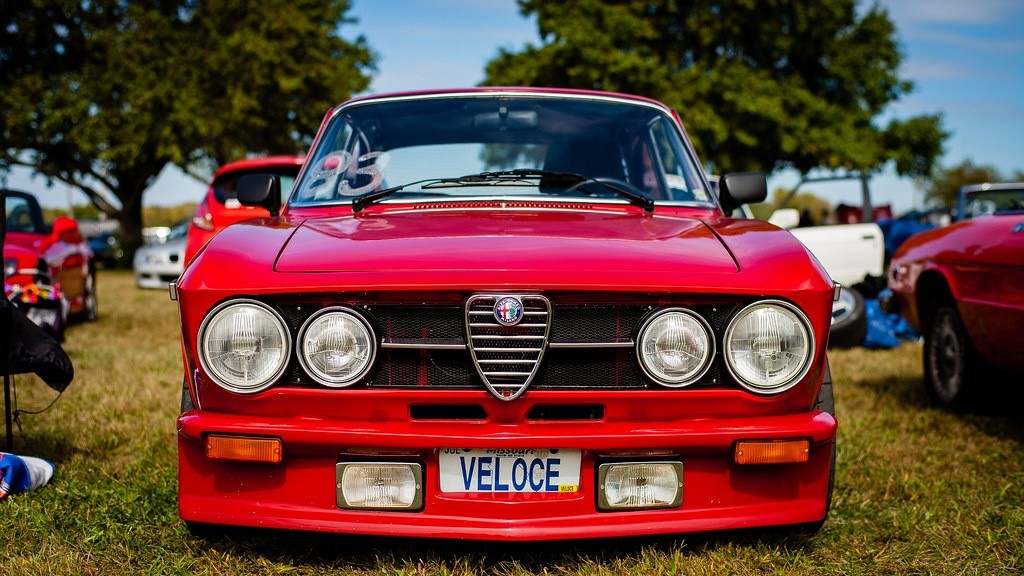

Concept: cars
[[2, 188, 97, 341], [69, 212, 126, 271], [887, 212, 1024, 418], [182, 153, 387, 271], [133, 216, 190, 290], [173, 85, 840, 542]]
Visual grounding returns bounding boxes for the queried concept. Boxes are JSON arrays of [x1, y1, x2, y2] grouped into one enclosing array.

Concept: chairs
[[539, 138, 629, 196], [5, 204, 36, 232]]
